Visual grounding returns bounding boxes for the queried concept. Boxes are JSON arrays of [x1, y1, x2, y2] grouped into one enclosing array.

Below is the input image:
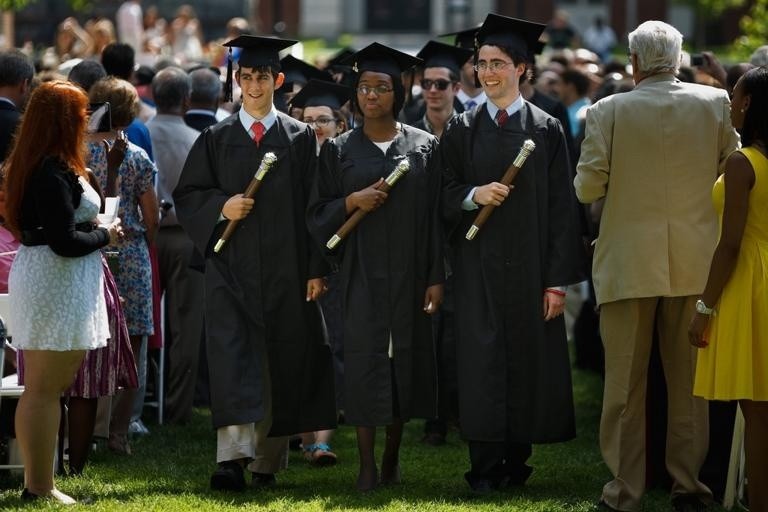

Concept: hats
[[222, 34, 299, 103], [286, 80, 355, 115], [416, 12, 547, 90], [341, 42, 423, 113], [280, 54, 332, 90]]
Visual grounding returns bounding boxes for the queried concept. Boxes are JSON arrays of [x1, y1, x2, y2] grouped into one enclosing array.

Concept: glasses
[[356, 85, 394, 95], [304, 117, 339, 127]]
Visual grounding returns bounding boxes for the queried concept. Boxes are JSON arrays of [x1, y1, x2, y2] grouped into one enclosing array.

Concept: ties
[[494, 109, 510, 131], [250, 121, 267, 145], [467, 100, 474, 113]]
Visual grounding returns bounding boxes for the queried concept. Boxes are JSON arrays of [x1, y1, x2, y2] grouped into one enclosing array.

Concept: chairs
[[0, 291, 70, 479]]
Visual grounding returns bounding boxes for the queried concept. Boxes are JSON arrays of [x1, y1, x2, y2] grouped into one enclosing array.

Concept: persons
[[0, 4, 768, 512]]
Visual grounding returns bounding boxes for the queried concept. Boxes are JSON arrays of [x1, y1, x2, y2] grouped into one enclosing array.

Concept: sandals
[[299, 440, 339, 466]]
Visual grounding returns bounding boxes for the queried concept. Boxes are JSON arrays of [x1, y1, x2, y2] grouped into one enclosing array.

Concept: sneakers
[[210, 462, 277, 492], [19, 486, 78, 506]]
